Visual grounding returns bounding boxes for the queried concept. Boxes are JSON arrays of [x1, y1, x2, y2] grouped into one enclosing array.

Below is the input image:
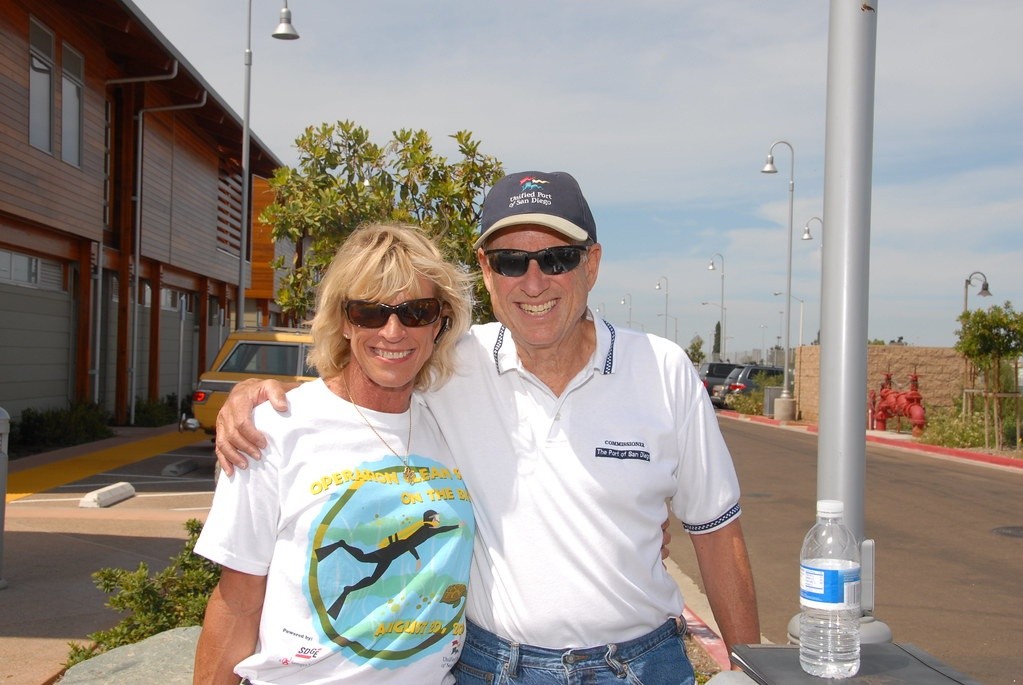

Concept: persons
[[215, 171, 760, 685], [193, 218, 671, 685]]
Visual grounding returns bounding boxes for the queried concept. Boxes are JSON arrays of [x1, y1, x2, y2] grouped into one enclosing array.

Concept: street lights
[[621, 292, 632, 329], [759, 142, 797, 421], [707, 252, 726, 362], [701, 301, 727, 361], [597, 302, 606, 320], [964, 271, 993, 311], [655, 276, 668, 338], [235, 1, 299, 328], [773, 291, 804, 347], [656, 312, 677, 343]]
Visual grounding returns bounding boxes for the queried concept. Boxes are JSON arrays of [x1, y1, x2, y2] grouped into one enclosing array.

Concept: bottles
[[798, 499, 862, 680]]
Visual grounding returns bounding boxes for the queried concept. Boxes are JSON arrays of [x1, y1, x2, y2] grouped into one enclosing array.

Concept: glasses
[[343, 298, 443, 328], [484, 245, 586, 278]]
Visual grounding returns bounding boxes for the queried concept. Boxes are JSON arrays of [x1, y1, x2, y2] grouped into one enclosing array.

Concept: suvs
[[720, 361, 785, 403], [698, 362, 739, 409]]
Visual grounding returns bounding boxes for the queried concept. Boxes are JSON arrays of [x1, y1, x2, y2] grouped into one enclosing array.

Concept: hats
[[473, 171, 597, 249]]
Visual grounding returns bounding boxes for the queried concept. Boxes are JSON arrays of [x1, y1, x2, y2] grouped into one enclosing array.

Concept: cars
[[177, 326, 319, 445]]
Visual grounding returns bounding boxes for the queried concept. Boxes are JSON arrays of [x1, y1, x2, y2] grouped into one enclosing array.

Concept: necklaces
[[341, 368, 418, 485]]
